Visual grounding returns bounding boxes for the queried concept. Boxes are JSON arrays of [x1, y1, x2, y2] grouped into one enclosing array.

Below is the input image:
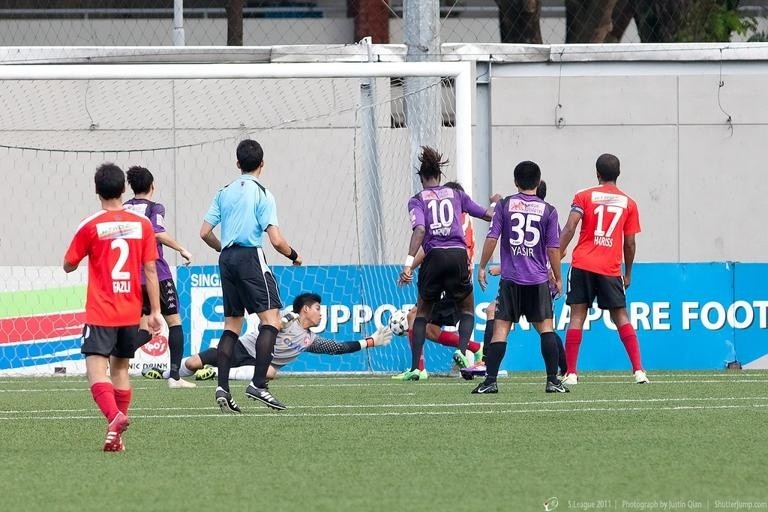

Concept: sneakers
[[544, 380, 569, 394], [631, 370, 649, 383], [472, 382, 499, 394], [392, 369, 419, 382], [245, 382, 286, 409], [216, 386, 240, 414], [474, 342, 483, 364], [562, 373, 577, 385], [454, 350, 470, 370], [420, 369, 427, 381], [104, 411, 126, 451], [168, 377, 196, 390], [141, 367, 165, 381], [196, 366, 214, 381]]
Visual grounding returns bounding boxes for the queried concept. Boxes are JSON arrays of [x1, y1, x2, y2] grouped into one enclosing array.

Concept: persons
[[122, 165, 199, 391], [63, 163, 162, 453], [471, 161, 570, 393], [142, 293, 393, 382], [393, 146, 504, 381], [392, 183, 484, 384], [200, 139, 302, 414], [460, 180, 567, 378], [559, 154, 651, 384]]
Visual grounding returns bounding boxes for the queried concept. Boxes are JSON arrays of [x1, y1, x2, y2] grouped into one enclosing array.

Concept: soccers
[[387, 308, 412, 336]]
